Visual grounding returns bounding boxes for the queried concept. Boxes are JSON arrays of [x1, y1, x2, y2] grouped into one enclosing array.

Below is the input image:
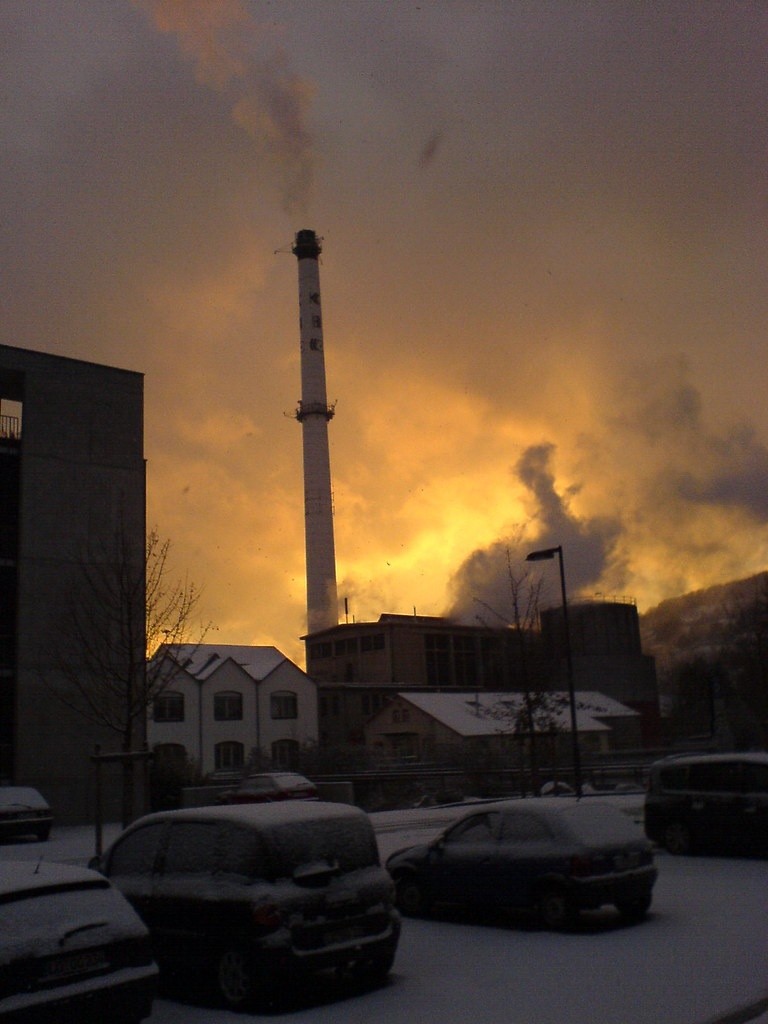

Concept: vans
[[643, 751, 768, 856], [87, 801, 402, 1010]]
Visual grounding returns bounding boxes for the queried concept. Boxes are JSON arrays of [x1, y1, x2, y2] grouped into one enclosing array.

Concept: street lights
[[524, 541, 592, 795]]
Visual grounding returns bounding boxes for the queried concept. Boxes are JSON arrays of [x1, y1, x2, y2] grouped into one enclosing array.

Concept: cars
[[388, 798, 655, 930], [604, 780, 643, 791], [0, 856, 158, 1024], [215, 772, 319, 806], [0, 787, 55, 842]]
